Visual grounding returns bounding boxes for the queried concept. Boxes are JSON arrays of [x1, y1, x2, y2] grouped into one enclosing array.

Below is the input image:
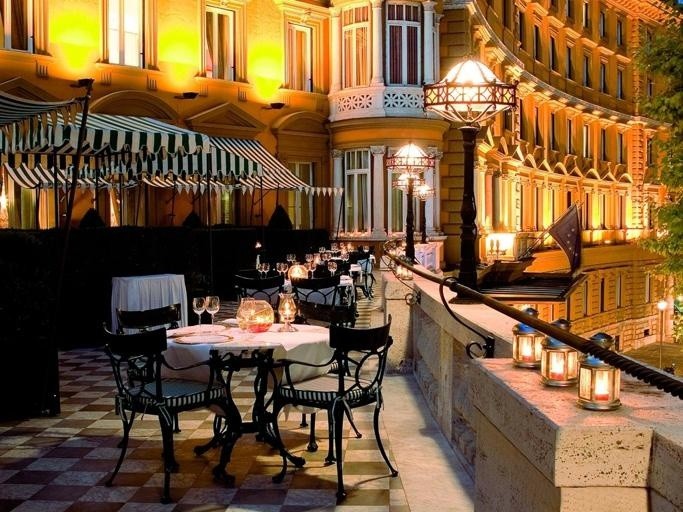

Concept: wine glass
[[286, 253, 296, 263], [240, 296, 255, 334], [275, 262, 289, 282], [257, 262, 270, 279], [192, 297, 206, 335], [305, 242, 350, 279], [205, 295, 221, 335]]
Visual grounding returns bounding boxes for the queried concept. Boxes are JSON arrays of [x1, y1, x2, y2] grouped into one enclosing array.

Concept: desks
[[110, 274, 188, 335]]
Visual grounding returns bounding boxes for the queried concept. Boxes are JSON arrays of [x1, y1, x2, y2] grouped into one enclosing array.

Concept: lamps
[[392, 255, 413, 280], [423, 53, 516, 304], [512, 308, 622, 411], [385, 140, 436, 264]]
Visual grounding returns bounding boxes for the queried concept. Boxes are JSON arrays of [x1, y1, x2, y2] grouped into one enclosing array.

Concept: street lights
[[656, 298, 668, 370], [385, 138, 437, 262], [413, 48, 523, 309]]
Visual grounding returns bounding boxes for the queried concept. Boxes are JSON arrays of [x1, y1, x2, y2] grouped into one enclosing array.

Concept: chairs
[[233, 241, 375, 328]]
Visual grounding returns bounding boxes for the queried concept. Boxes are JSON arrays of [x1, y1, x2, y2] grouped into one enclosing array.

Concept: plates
[[174, 318, 237, 345]]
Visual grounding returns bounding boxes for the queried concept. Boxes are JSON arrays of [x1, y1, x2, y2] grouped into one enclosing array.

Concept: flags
[[546, 203, 583, 277]]
